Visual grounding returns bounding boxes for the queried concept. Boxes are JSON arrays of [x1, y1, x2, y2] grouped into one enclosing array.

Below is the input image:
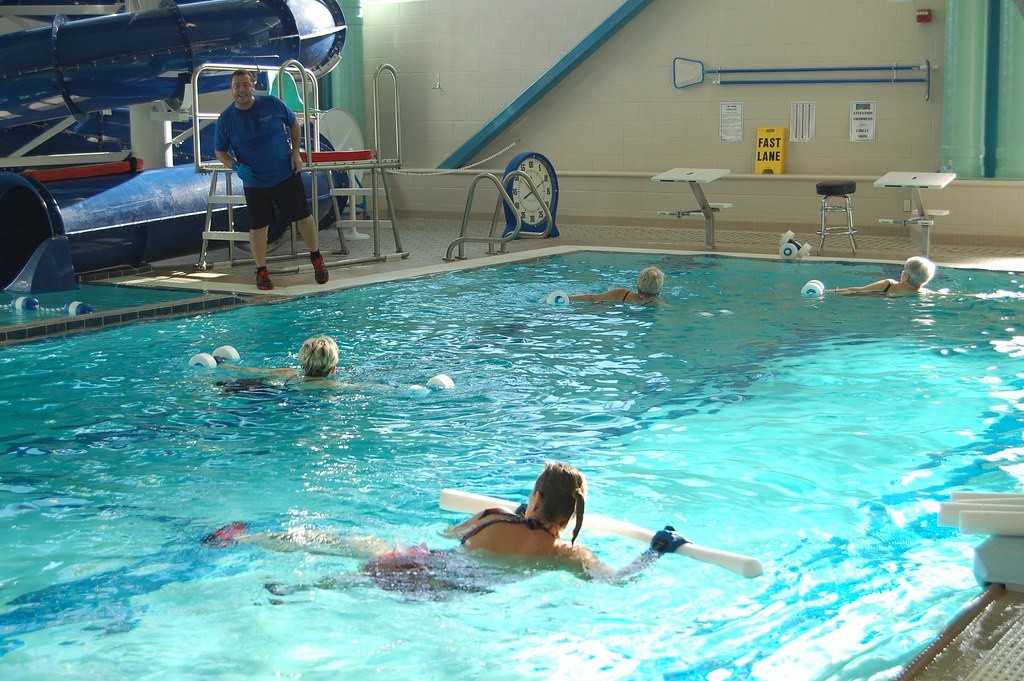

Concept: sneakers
[[256, 269, 274, 290], [310, 255, 329, 284]]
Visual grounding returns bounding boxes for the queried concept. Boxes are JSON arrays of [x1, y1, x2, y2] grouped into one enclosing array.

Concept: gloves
[[644, 525, 691, 557], [232, 162, 252, 183]]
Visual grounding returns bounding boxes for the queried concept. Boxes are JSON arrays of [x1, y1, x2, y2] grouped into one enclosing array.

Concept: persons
[[823, 256, 936, 293], [215, 69, 329, 290], [299, 335, 339, 376], [568, 267, 669, 305], [458, 460, 693, 556]]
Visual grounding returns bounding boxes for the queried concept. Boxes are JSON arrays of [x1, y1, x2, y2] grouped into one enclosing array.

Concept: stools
[[814, 180, 860, 253]]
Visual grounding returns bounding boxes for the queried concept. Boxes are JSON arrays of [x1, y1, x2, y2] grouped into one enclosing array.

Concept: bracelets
[[835, 289, 838, 293]]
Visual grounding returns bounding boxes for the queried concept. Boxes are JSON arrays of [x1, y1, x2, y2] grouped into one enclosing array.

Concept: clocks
[[501, 152, 561, 239]]
